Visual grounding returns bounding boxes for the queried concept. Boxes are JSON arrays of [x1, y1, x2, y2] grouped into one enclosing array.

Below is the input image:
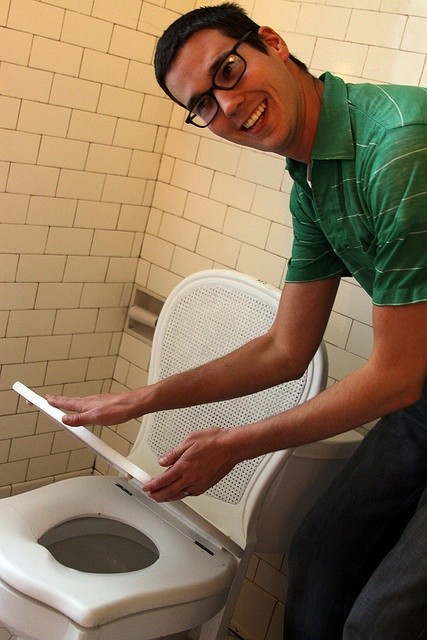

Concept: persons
[[47, 1, 427, 639]]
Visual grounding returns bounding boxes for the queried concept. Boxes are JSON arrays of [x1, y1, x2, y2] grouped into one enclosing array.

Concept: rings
[[184, 492, 194, 500]]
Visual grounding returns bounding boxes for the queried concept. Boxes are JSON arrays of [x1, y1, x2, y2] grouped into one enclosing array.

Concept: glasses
[[185, 30, 254, 128]]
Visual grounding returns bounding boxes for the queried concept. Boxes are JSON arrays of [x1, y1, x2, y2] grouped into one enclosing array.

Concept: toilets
[[0, 268, 365, 640]]
